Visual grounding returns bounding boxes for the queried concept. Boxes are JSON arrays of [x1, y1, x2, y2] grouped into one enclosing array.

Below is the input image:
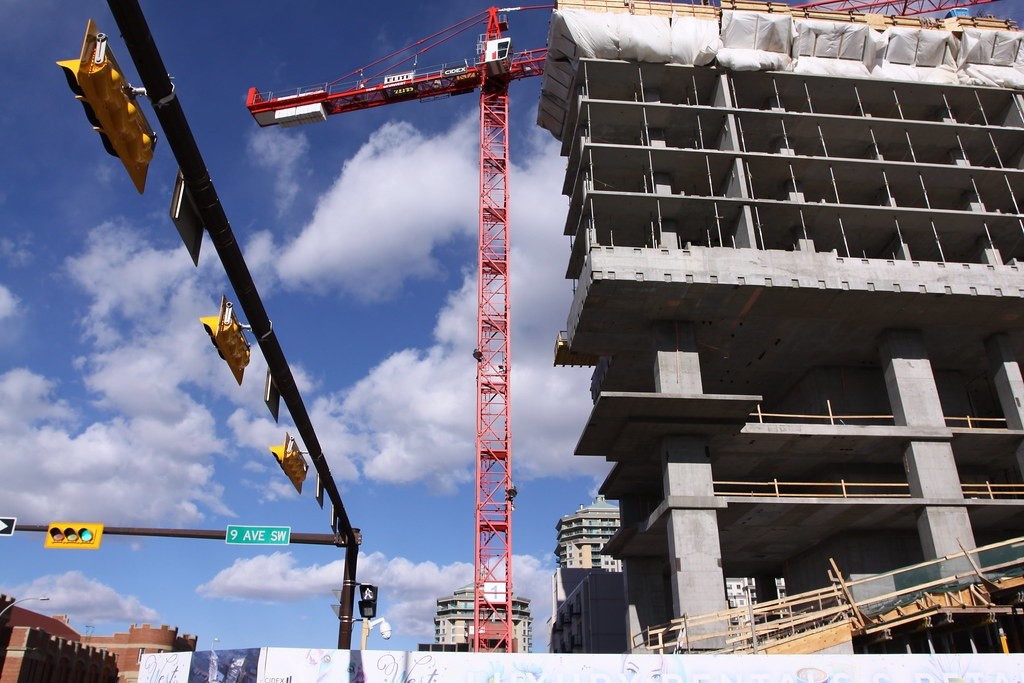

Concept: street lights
[[211, 638, 220, 650], [361, 617, 392, 650], [0, 596, 50, 617]]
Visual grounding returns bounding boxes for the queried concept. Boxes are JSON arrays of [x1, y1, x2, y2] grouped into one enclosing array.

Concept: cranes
[[246, 0, 1002, 653]]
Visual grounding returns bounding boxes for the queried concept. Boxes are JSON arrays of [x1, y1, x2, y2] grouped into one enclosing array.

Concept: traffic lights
[[357, 584, 378, 618], [43, 522, 104, 549], [269, 432, 310, 494], [198, 294, 250, 385], [55, 20, 158, 196]]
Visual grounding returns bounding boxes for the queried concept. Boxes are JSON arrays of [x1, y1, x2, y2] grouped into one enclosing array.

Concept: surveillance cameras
[[380, 622, 392, 640]]
